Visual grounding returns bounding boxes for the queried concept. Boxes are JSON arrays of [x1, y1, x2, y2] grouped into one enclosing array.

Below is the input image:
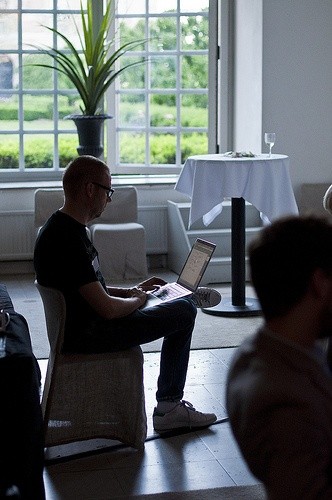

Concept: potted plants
[[20, 1, 162, 162]]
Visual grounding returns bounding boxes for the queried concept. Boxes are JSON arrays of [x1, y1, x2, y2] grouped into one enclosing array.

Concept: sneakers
[[188, 287, 221, 308], [153, 400, 217, 430]]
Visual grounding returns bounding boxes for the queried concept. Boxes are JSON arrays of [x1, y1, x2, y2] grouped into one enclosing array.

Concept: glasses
[[84, 182, 114, 198]]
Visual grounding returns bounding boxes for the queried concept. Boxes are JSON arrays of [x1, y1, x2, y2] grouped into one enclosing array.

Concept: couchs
[[0, 284, 47, 500]]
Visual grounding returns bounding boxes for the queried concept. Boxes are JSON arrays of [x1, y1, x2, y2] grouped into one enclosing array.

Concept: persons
[[33, 155, 221, 434], [227, 213, 331, 500]]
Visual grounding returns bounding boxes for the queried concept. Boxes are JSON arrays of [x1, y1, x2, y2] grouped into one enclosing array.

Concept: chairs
[[88, 185, 148, 281], [32, 186, 92, 285], [34, 280, 148, 457]]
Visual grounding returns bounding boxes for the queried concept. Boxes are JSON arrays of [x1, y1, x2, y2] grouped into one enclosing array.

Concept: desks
[[174, 154, 299, 307]]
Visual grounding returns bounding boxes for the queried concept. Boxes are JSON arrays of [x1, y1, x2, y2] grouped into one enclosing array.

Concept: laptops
[[138, 237, 216, 311]]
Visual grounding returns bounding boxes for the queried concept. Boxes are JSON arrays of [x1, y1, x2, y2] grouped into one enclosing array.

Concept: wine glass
[[264, 132, 276, 158]]
